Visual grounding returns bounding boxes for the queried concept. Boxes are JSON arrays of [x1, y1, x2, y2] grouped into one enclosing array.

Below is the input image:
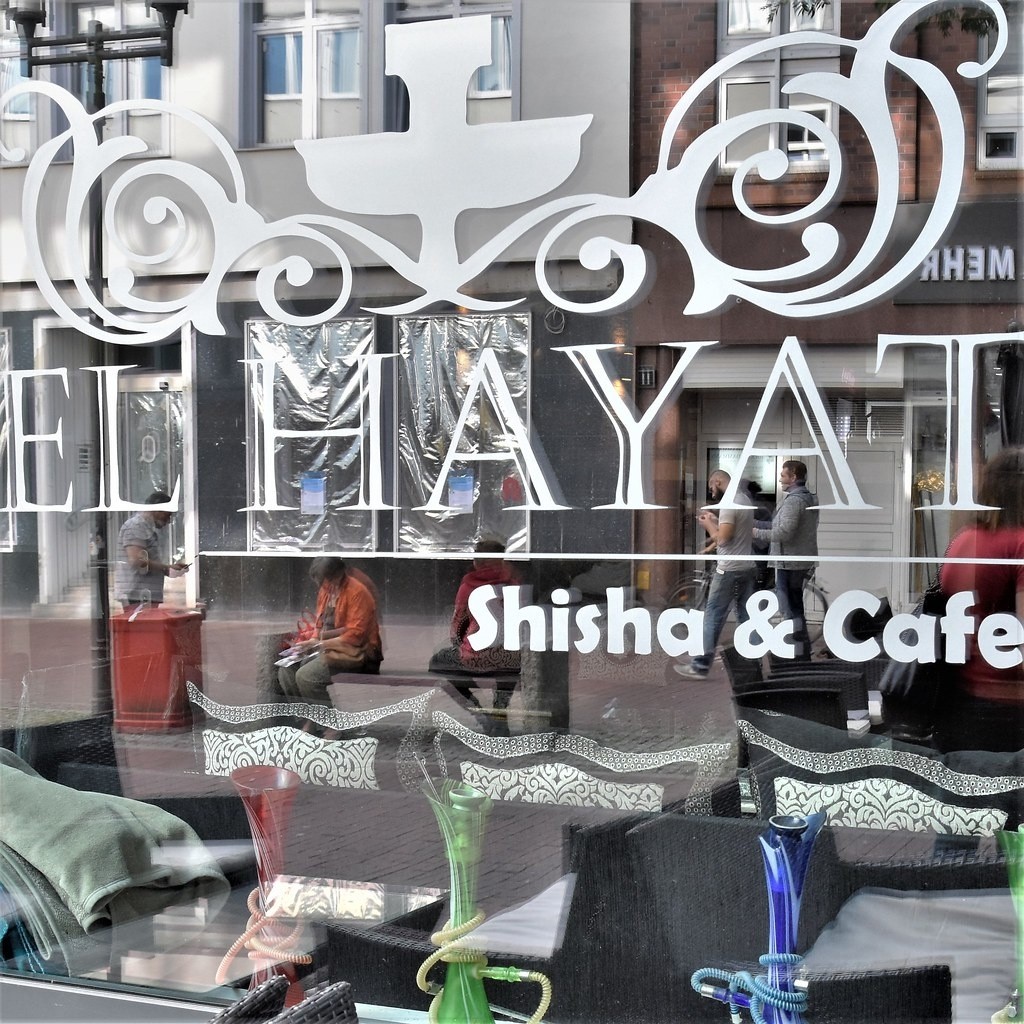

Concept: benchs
[[329, 672, 521, 735], [0, 718, 262, 963], [435, 780, 1024, 1024]]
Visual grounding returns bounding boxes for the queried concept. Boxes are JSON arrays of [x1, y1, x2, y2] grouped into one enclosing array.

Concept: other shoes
[[300, 718, 318, 736], [673, 663, 706, 680], [322, 729, 342, 740], [478, 712, 509, 737]]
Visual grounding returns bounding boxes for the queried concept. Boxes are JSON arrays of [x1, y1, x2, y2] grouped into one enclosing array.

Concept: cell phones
[[185, 563, 193, 569]]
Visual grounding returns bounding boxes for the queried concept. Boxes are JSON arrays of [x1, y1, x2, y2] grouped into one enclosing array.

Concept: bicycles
[[662, 551, 831, 646]]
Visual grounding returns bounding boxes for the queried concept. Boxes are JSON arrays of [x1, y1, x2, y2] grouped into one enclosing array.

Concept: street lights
[[2, 1, 192, 766]]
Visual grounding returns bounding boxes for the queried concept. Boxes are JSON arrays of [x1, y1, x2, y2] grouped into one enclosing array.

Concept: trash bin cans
[[108, 606, 208, 737]]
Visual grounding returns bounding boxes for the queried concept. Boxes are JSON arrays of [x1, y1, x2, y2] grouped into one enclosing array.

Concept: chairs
[[718, 618, 877, 776]]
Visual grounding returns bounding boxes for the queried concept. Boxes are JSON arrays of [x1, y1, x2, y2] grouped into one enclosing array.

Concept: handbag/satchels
[[867, 530, 968, 737], [295, 611, 319, 644]]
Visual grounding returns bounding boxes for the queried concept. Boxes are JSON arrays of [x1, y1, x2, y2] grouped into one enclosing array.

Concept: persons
[[427, 540, 529, 736], [115, 492, 190, 613], [752, 460, 820, 662], [275, 543, 383, 743], [674, 469, 763, 680], [932, 447, 1024, 851]]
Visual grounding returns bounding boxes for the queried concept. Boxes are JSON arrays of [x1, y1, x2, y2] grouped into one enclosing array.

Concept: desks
[[0, 873, 451, 1024]]
